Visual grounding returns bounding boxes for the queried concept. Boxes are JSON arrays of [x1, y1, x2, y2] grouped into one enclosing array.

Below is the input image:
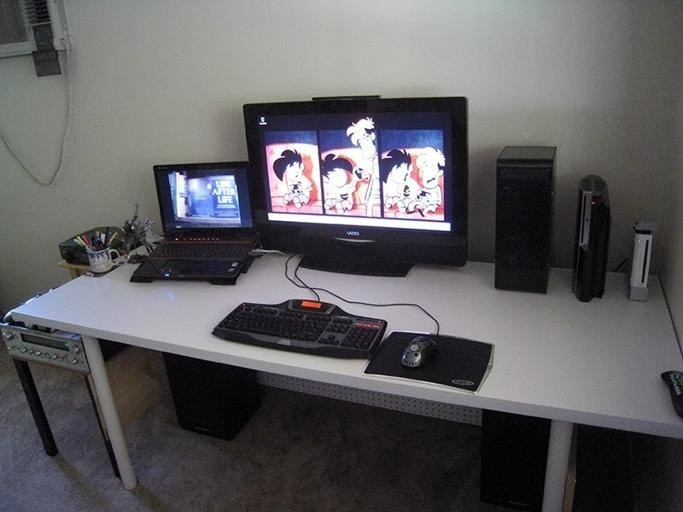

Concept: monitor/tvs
[[246, 95, 468, 279]]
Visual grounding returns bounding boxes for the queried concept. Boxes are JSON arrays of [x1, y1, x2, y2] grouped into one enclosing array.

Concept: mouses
[[402, 336, 438, 368]]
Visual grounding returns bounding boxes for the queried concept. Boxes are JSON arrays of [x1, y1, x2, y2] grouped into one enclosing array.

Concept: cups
[[87, 248, 119, 274]]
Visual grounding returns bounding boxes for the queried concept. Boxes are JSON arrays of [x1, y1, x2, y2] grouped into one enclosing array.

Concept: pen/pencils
[[73, 226, 117, 251]]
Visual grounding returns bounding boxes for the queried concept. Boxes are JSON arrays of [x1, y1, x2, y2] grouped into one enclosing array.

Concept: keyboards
[[210, 301, 388, 358]]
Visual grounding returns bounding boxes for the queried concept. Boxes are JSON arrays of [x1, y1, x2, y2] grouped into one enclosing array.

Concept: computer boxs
[[495, 147, 555, 292]]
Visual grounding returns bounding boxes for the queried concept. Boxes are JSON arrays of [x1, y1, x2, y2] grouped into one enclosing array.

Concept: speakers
[[160, 353, 261, 442], [478, 409, 552, 512]]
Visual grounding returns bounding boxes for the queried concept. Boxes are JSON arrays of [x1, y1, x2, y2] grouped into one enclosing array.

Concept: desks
[[9, 228, 683, 512]]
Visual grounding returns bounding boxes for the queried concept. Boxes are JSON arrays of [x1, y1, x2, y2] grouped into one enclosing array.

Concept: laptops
[[128, 162, 260, 286]]
